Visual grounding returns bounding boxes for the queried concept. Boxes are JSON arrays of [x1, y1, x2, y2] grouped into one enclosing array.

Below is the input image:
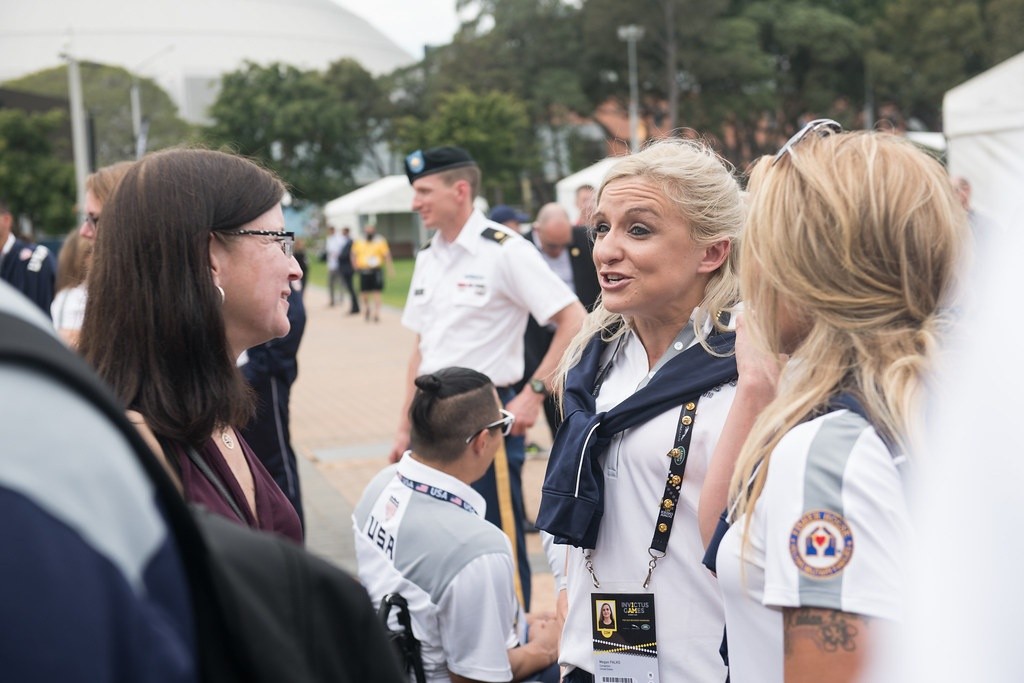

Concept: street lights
[[57, 48, 93, 223], [616, 23, 650, 156]]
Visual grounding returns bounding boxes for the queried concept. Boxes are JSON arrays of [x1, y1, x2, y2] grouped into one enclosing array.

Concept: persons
[[698, 128, 971, 682], [532, 141, 747, 683], [235, 240, 309, 523], [326, 224, 397, 322], [50, 162, 138, 346], [0, 199, 57, 318], [489, 187, 603, 458], [352, 367, 562, 683], [388, 145, 589, 612], [599, 603, 615, 628], [77, 148, 304, 544], [0, 279, 196, 683]]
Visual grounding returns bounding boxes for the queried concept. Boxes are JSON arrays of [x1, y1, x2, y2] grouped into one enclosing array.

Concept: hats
[[491, 206, 529, 223], [405, 147, 476, 183]]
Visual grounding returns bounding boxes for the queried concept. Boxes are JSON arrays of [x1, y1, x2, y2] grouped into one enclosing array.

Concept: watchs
[[529, 379, 548, 395]]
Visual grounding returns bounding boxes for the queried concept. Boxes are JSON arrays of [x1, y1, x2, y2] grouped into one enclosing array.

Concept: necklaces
[[218, 428, 234, 448]]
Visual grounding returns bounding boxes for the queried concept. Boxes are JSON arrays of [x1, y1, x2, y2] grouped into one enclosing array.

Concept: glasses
[[770, 119, 842, 167], [465, 410, 516, 443], [85, 216, 98, 233], [217, 230, 296, 258]]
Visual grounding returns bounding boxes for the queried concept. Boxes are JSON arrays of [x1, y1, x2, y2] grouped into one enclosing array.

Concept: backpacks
[[0, 311, 401, 683]]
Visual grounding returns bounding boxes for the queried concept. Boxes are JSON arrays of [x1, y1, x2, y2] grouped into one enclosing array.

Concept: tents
[[943, 51, 1024, 239], [324, 175, 486, 255]]
